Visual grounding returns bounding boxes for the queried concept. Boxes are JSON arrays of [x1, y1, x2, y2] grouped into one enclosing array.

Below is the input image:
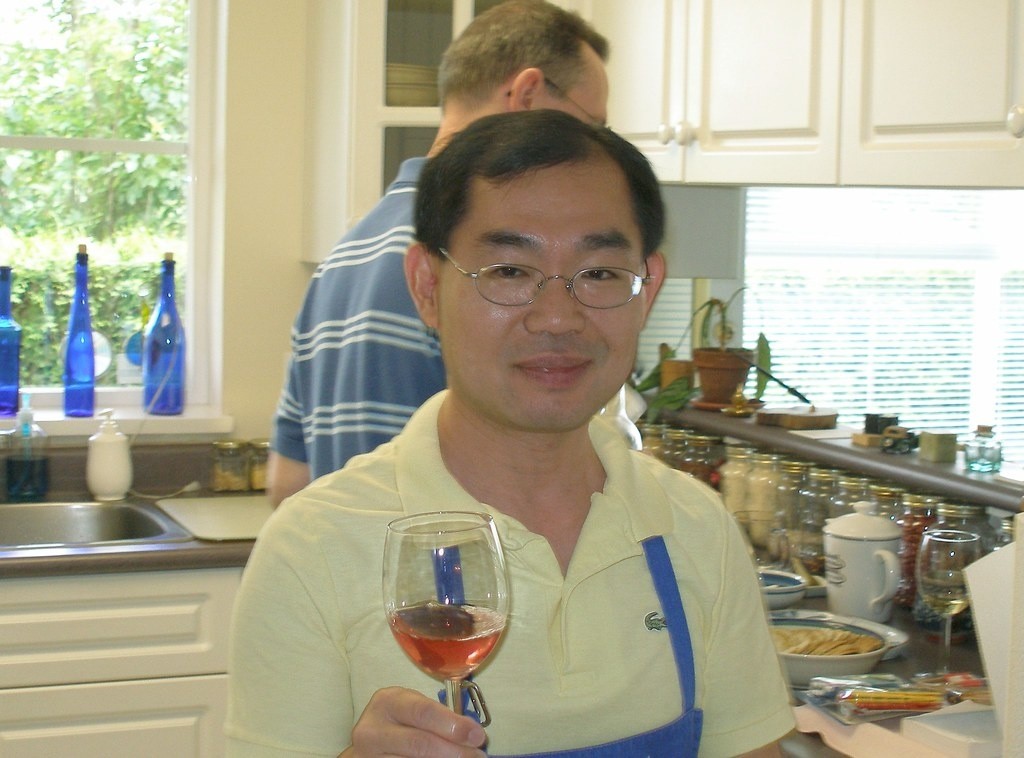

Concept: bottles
[[212, 439, 271, 492], [635, 423, 1012, 627], [0, 265, 21, 416], [964, 424, 1001, 472], [143, 252, 187, 417], [64, 244, 96, 418]]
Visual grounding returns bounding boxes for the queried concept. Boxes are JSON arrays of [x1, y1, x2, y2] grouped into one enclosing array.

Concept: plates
[[814, 609, 911, 659]]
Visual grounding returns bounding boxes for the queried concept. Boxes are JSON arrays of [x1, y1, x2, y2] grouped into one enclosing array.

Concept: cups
[[819, 498, 903, 624]]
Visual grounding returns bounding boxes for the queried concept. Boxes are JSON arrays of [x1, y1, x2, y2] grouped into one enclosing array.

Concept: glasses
[[505, 73, 613, 133], [434, 245, 654, 310]]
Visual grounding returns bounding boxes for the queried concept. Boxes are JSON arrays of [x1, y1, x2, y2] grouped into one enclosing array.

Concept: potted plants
[[632, 286, 810, 422]]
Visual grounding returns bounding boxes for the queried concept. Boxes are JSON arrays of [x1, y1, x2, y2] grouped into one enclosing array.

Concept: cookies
[[763, 627, 883, 655]]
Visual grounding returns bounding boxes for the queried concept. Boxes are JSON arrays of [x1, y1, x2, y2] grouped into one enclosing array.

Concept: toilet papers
[[1003, 511, 1024, 758]]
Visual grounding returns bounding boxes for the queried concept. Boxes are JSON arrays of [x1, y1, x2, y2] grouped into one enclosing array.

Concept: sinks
[[0, 502, 166, 546]]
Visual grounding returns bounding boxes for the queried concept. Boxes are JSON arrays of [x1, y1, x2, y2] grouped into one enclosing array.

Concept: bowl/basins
[[765, 618, 888, 689], [755, 566, 804, 611]]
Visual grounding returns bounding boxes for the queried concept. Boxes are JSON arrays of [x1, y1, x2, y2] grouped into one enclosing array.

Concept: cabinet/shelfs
[[300, 0, 1024, 266], [0, 565, 242, 758]]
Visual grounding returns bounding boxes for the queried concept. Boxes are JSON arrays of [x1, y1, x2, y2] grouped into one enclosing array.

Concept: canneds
[[207, 438, 270, 492]]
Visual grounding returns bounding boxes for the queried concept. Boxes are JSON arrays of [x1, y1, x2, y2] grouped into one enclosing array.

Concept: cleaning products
[[3, 391, 51, 502], [85, 408, 135, 501]]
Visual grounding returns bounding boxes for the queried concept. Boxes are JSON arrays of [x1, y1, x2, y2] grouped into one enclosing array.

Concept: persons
[[266, 1, 608, 512], [225, 107, 799, 758]]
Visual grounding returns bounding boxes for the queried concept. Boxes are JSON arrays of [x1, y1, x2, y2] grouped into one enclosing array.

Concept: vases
[[661, 359, 694, 395]]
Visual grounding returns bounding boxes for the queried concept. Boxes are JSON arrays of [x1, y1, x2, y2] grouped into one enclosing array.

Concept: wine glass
[[381, 509, 512, 721], [916, 528, 981, 683]]
